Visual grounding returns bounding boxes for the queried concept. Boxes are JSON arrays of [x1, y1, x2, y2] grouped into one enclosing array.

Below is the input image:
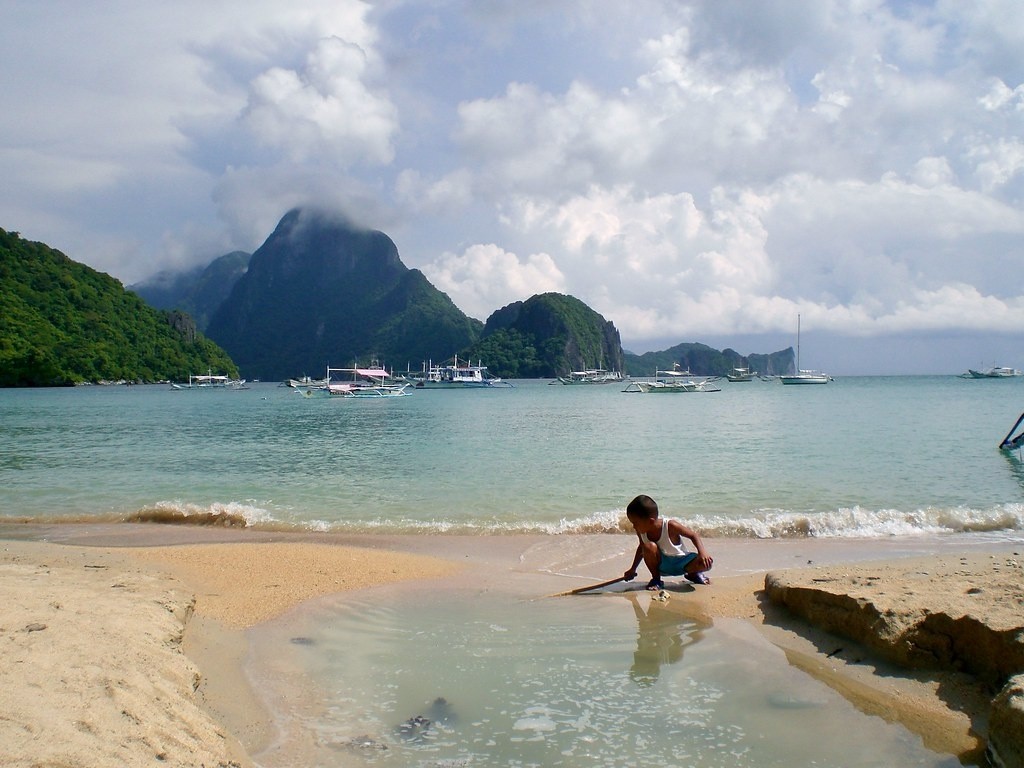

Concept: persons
[[625, 494, 713, 590]]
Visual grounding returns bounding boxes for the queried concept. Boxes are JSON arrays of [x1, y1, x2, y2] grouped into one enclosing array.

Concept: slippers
[[684, 572, 709, 584], [646, 579, 664, 591]]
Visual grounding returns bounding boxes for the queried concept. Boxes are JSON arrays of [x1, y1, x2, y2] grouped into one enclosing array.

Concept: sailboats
[[780, 314, 828, 385]]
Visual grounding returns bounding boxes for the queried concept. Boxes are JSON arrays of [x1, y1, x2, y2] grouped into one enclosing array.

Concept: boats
[[548, 360, 625, 385], [969, 367, 1023, 378], [283, 374, 332, 387], [170, 370, 246, 390], [324, 368, 399, 395], [727, 367, 756, 382], [402, 355, 502, 388], [621, 362, 720, 393]]
